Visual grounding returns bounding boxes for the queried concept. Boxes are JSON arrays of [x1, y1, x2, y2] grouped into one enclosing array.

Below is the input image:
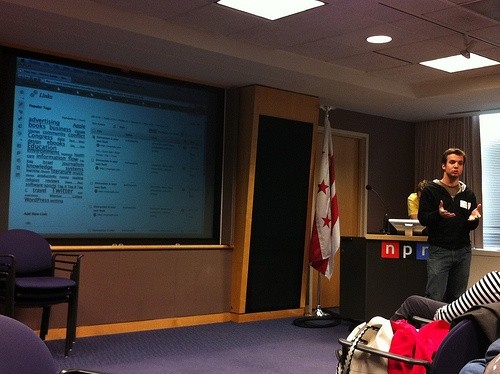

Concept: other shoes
[[335, 349, 343, 361]]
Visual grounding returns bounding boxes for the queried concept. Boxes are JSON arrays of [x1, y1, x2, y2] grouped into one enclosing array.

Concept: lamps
[[460, 34, 478, 58]]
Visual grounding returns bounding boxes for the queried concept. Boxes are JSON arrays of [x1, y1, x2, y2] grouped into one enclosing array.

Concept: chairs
[[0, 228, 101, 374], [339, 301, 500, 374]]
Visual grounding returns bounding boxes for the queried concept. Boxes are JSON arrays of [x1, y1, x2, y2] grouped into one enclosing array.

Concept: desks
[[340, 234, 428, 330]]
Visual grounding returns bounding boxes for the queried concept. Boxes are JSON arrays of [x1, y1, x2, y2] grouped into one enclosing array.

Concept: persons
[[407, 180, 428, 219], [334, 271, 500, 362], [418, 148, 481, 304]]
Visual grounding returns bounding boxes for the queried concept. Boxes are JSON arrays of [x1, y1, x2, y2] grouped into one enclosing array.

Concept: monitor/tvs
[[388, 218, 426, 232]]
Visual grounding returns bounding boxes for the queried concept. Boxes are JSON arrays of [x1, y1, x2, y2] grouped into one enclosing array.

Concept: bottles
[[383, 214, 390, 234]]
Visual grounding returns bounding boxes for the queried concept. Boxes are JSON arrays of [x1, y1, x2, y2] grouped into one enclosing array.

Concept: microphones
[[366, 185, 389, 234]]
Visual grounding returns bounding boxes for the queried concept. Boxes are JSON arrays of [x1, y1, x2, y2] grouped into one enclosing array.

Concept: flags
[[308, 119, 340, 280]]
